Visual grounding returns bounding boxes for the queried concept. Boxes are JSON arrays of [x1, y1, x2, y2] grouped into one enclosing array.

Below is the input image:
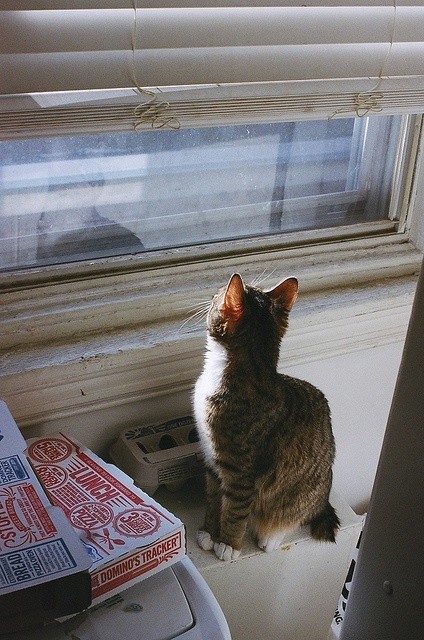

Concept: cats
[[175, 267, 342, 564]]
